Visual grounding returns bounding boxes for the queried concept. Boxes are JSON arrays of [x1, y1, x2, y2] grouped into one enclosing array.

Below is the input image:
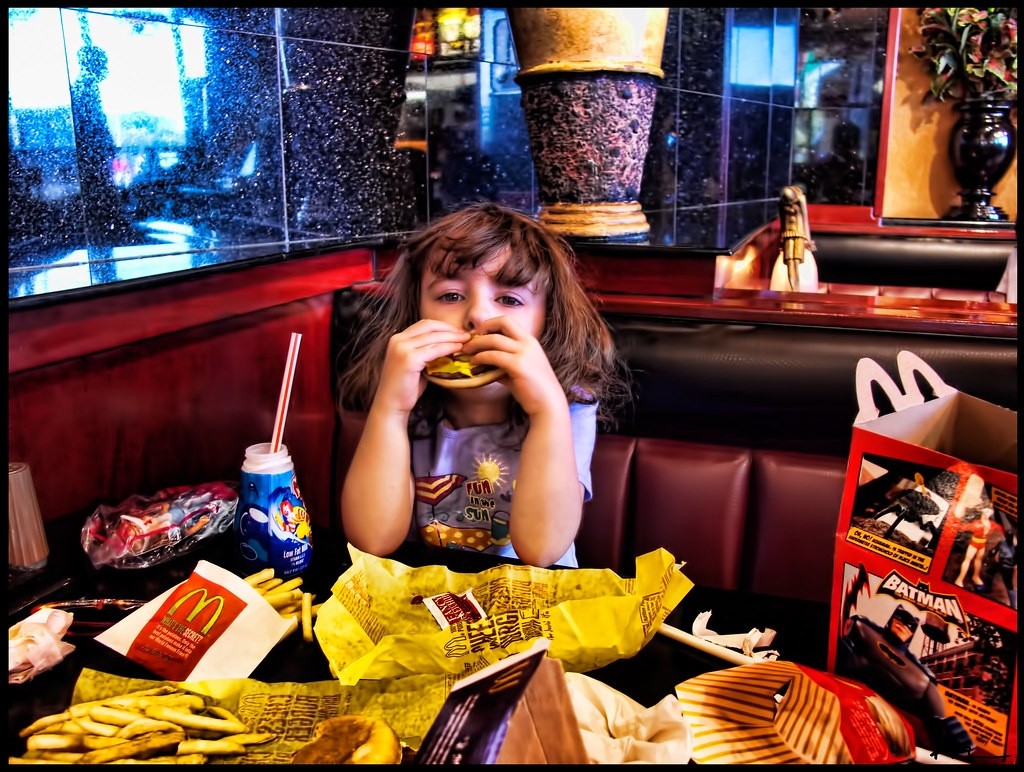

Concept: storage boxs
[[415, 637, 589, 765], [827, 344, 1020, 763]]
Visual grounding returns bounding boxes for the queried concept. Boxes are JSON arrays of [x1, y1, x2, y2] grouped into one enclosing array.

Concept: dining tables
[[8, 499, 829, 765]]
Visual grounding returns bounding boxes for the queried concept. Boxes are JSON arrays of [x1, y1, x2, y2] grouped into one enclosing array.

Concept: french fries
[[243, 567, 325, 643], [8, 685, 278, 764]]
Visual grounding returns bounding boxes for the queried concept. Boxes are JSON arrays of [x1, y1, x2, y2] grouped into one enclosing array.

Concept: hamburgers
[[421, 333, 506, 389]]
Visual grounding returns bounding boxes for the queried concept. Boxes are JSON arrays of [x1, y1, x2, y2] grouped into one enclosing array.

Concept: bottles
[[234, 442, 312, 580]]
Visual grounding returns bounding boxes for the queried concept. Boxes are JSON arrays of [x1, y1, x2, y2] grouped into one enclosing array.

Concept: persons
[[334, 201, 631, 570]]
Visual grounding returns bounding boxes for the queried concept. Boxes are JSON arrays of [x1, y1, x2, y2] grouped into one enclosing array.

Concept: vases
[[943, 101, 1017, 221]]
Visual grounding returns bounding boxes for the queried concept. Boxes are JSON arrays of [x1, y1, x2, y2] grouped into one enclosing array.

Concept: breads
[[286, 714, 401, 765]]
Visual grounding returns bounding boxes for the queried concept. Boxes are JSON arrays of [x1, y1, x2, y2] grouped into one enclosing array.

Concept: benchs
[[334, 226, 1018, 602]]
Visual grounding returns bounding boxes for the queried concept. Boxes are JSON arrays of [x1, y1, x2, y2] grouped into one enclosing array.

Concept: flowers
[[907, 8, 1017, 105]]
